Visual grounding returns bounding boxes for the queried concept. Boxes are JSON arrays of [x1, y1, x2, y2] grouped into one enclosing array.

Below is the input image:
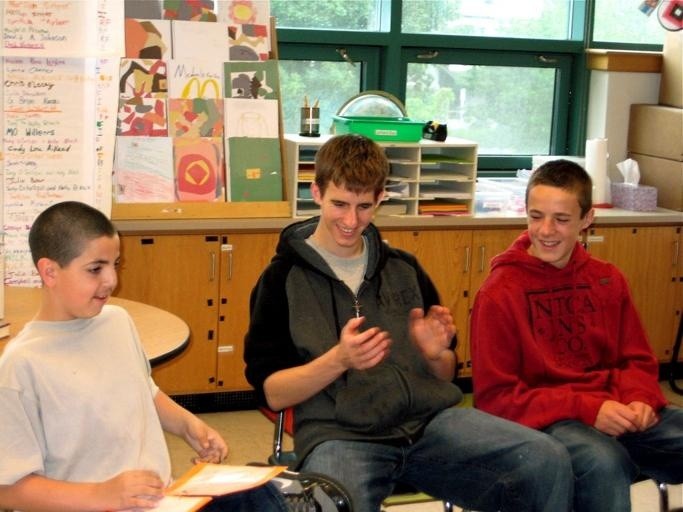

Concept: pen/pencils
[[304, 95, 308, 107], [314, 94, 321, 108]]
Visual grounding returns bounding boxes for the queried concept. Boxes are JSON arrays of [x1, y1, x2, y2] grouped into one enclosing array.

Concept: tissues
[[612, 158, 658, 212]]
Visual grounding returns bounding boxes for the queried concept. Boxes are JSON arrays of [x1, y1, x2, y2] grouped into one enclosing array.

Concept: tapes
[[429, 122, 438, 132]]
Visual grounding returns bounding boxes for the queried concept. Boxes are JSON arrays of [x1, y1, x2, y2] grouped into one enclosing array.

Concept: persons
[[243, 134, 574, 512], [0, 201, 289, 512], [470, 159, 683, 512]]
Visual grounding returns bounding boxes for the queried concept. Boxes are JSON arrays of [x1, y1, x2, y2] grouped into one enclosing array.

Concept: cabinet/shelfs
[[581, 226, 682, 372], [110, 232, 282, 413], [381, 228, 529, 377], [283, 134, 477, 218]]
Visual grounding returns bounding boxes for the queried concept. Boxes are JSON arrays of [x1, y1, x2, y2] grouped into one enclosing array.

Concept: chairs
[[244, 282, 455, 512]]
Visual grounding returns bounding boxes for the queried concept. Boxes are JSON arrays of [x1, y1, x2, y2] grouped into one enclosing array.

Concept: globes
[[330, 90, 408, 136]]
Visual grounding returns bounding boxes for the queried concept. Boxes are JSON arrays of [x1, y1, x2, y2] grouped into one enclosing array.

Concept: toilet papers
[[585, 138, 612, 206]]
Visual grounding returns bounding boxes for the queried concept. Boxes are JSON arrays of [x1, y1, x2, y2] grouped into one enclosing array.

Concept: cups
[[301, 106, 321, 134]]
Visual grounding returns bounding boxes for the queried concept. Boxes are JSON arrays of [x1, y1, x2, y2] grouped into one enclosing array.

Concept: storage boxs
[[626, 27, 683, 212]]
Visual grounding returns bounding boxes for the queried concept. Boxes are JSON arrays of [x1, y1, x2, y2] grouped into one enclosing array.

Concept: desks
[[0, 285, 191, 368]]
